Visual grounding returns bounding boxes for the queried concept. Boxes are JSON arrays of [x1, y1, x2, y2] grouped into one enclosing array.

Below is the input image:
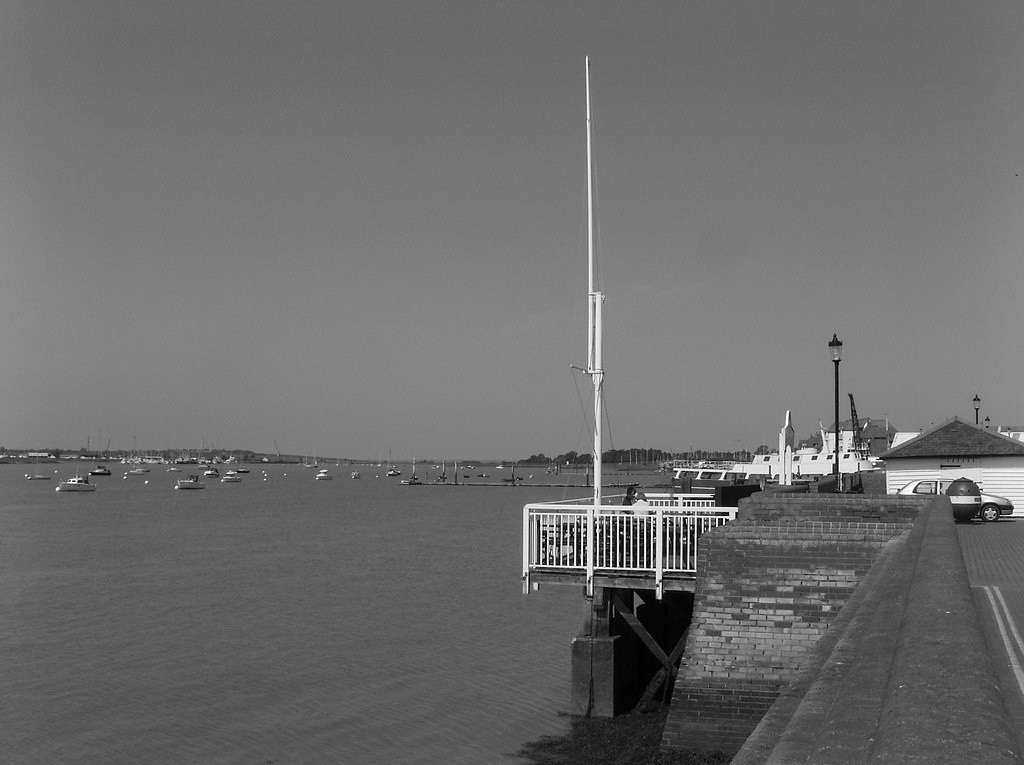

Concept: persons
[[425, 473, 428, 482], [737, 475, 744, 485], [632, 492, 649, 518], [622, 487, 637, 515]]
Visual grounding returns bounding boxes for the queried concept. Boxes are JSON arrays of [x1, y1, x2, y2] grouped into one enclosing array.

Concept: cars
[[895, 479, 1014, 523]]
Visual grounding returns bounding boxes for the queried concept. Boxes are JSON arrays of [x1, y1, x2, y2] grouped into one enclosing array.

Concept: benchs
[[541, 519, 700, 569]]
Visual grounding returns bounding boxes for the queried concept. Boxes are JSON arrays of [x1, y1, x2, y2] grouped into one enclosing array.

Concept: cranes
[[847, 393, 864, 452]]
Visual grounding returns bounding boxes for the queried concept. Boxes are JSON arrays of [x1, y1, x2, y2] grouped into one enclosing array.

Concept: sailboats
[[33, 450, 52, 480]]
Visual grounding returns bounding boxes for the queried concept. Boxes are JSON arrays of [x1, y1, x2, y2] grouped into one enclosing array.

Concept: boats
[[303, 445, 505, 480], [204, 466, 220, 478], [166, 467, 183, 472], [223, 469, 241, 482], [121, 435, 238, 464], [128, 468, 150, 475], [177, 474, 206, 490], [88, 465, 112, 476], [197, 464, 211, 470], [236, 466, 250, 473], [59, 459, 97, 491]]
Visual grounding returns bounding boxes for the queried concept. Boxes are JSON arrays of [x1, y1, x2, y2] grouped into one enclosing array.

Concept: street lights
[[972, 394, 981, 425], [828, 333, 843, 475], [1006, 426, 1011, 437]]
